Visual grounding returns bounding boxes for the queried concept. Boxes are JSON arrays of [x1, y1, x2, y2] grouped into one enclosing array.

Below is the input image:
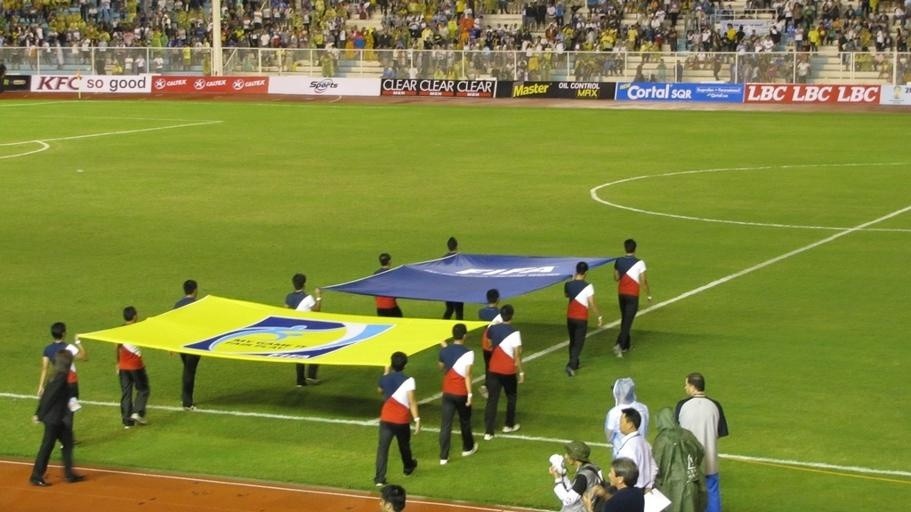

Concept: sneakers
[[374, 480, 390, 488], [502, 423, 522, 433], [124, 423, 133, 431], [612, 344, 637, 361], [129, 412, 149, 424], [305, 376, 322, 385], [439, 458, 449, 466], [403, 456, 418, 477], [296, 380, 310, 389], [183, 405, 196, 411], [478, 384, 490, 400], [564, 359, 580, 378], [461, 441, 480, 457], [484, 433, 495, 440]]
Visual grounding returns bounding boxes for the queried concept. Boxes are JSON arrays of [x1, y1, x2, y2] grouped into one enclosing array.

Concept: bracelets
[[412, 418, 419, 422], [315, 297, 321, 301], [468, 393, 473, 396], [74, 340, 81, 345], [598, 317, 602, 320], [647, 297, 652, 299], [518, 373, 523, 376]]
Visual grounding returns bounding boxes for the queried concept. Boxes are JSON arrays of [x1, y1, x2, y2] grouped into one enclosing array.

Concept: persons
[[37, 323, 86, 447], [379, 485, 406, 512], [443, 238, 464, 319], [169, 279, 200, 410], [115, 307, 149, 429], [484, 304, 525, 441], [613, 239, 652, 359], [549, 372, 729, 512], [375, 351, 420, 487], [284, 274, 322, 388], [437, 322, 479, 465], [479, 289, 501, 398], [30, 349, 84, 486], [373, 253, 402, 316], [564, 261, 602, 376]]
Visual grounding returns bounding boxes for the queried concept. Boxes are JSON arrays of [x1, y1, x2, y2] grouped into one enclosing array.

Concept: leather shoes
[[65, 474, 87, 482], [30, 477, 52, 487]]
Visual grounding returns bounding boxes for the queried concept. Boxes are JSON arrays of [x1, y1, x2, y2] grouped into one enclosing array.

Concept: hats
[[562, 439, 593, 465]]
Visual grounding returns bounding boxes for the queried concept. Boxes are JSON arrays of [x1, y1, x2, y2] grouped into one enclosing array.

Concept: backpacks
[[575, 466, 618, 512]]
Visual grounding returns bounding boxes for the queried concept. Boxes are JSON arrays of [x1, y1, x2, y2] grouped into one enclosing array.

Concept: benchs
[[0, 0, 911, 87]]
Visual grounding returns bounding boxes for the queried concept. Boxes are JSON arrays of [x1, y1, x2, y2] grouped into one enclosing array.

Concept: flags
[[77, 294, 488, 369], [320, 250, 618, 304]]
